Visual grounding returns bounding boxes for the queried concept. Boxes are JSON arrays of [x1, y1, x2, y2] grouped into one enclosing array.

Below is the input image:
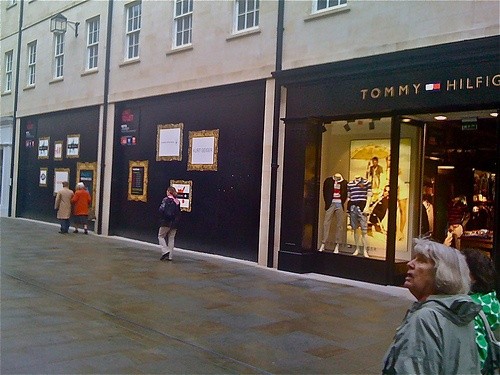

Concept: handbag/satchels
[[478, 310, 500, 375]]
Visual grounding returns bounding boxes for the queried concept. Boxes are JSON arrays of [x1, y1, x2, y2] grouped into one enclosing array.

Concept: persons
[[158, 187, 180, 262], [72, 183, 92, 234], [54, 181, 73, 234], [386, 155, 407, 240], [463, 250, 500, 374], [318, 173, 347, 255], [443, 217, 464, 249], [382, 240, 481, 375], [365, 156, 383, 191], [472, 205, 485, 230], [369, 187, 390, 229], [347, 176, 372, 259]]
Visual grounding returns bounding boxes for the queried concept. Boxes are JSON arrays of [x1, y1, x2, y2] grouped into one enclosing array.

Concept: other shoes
[[160, 251, 169, 260], [168, 259, 172, 261], [58, 228, 88, 234]]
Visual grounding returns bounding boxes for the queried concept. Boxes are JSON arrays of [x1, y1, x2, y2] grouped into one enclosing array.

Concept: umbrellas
[[349, 145, 393, 160]]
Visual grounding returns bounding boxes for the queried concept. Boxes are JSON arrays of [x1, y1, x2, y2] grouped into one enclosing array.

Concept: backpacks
[[163, 196, 176, 220]]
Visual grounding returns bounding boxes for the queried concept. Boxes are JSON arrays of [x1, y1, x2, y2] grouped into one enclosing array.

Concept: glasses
[[384, 189, 390, 193]]
